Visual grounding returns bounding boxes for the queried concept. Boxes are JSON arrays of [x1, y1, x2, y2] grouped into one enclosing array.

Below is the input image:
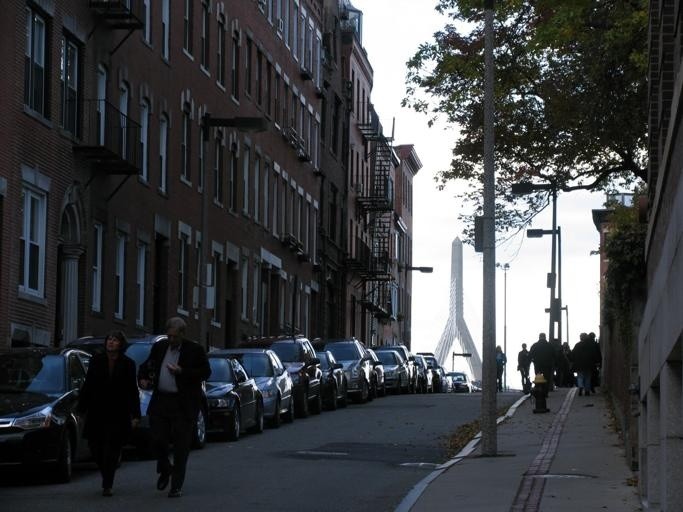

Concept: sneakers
[[578, 386, 596, 397], [102, 488, 113, 497]]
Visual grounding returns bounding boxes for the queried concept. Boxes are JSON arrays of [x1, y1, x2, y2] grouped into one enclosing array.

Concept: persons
[[74, 328, 142, 496], [517, 331, 601, 396], [136, 314, 208, 497], [496, 346, 507, 392]]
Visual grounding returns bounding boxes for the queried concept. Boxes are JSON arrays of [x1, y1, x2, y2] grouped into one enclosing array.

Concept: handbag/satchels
[[137, 366, 157, 390]]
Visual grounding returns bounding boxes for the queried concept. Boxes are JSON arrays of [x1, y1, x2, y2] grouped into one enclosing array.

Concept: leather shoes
[[157, 473, 169, 491], [167, 487, 184, 498]]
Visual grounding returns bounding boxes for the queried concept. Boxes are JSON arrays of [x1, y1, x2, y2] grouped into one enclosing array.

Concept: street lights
[[509, 178, 558, 342], [542, 304, 568, 344], [400, 264, 432, 345], [496, 263, 509, 393], [525, 223, 563, 344], [197, 108, 269, 352]]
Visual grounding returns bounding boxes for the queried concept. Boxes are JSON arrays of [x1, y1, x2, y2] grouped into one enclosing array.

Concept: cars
[[471, 379, 484, 392]]
